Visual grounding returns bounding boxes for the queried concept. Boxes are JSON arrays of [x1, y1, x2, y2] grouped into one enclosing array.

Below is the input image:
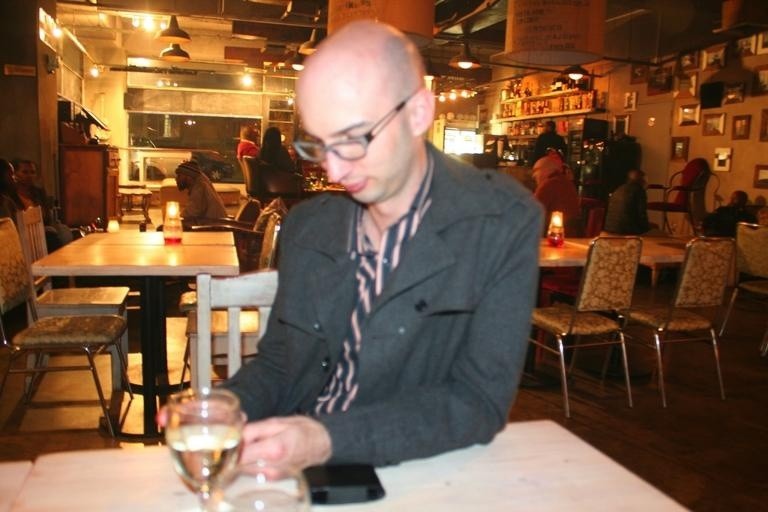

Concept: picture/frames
[[613, 33, 767, 189]]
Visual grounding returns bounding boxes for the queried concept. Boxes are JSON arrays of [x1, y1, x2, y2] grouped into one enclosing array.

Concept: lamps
[[159, 41, 190, 62], [447, 35, 482, 71], [289, 49, 306, 71], [562, 64, 587, 82], [281, 28, 309, 43], [158, 14, 192, 43]]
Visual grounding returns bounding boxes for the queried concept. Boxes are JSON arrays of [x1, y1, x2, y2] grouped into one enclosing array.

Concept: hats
[[175, 158, 202, 179]]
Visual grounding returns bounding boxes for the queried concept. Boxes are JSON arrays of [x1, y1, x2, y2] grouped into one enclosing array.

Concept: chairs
[[143, 157, 189, 208]]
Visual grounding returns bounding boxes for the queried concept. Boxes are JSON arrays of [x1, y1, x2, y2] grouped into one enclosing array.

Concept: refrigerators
[[568, 117, 608, 166]]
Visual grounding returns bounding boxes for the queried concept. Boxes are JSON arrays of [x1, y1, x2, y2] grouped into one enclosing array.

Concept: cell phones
[[303, 463, 385, 506]]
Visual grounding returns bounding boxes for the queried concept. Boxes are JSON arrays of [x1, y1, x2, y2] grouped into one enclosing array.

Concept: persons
[[531, 122, 566, 164], [604, 168, 650, 234], [0, 159, 24, 216], [700, 191, 757, 236], [175, 160, 228, 218], [237, 126, 261, 160], [530, 150, 581, 229], [15, 158, 73, 249], [157, 21, 545, 473], [260, 128, 295, 173]]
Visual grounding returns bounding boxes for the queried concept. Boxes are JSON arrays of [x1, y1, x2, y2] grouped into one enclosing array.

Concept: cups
[[222, 463, 308, 512]]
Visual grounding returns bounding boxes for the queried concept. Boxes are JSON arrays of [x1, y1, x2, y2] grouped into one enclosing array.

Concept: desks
[[118, 189, 153, 224], [10, 416, 693, 511], [0, 460, 31, 512]]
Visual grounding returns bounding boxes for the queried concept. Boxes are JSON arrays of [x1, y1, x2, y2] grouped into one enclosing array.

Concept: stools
[[161, 178, 181, 224]]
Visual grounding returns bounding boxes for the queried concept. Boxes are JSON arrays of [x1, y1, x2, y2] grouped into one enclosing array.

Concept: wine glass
[[164, 387, 245, 512]]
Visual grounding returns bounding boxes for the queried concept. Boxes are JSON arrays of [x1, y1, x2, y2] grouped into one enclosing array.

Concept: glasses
[[289, 87, 421, 163]]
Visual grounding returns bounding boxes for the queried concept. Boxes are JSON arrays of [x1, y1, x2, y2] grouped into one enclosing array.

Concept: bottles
[[501, 75, 606, 138], [164, 202, 183, 244], [547, 211, 565, 248]]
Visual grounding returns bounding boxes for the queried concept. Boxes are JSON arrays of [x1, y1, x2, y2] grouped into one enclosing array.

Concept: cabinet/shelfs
[[59, 144, 121, 229], [497, 88, 606, 168], [264, 90, 349, 194]]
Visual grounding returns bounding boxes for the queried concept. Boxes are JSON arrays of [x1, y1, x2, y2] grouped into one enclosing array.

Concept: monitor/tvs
[[443, 125, 485, 157]]
